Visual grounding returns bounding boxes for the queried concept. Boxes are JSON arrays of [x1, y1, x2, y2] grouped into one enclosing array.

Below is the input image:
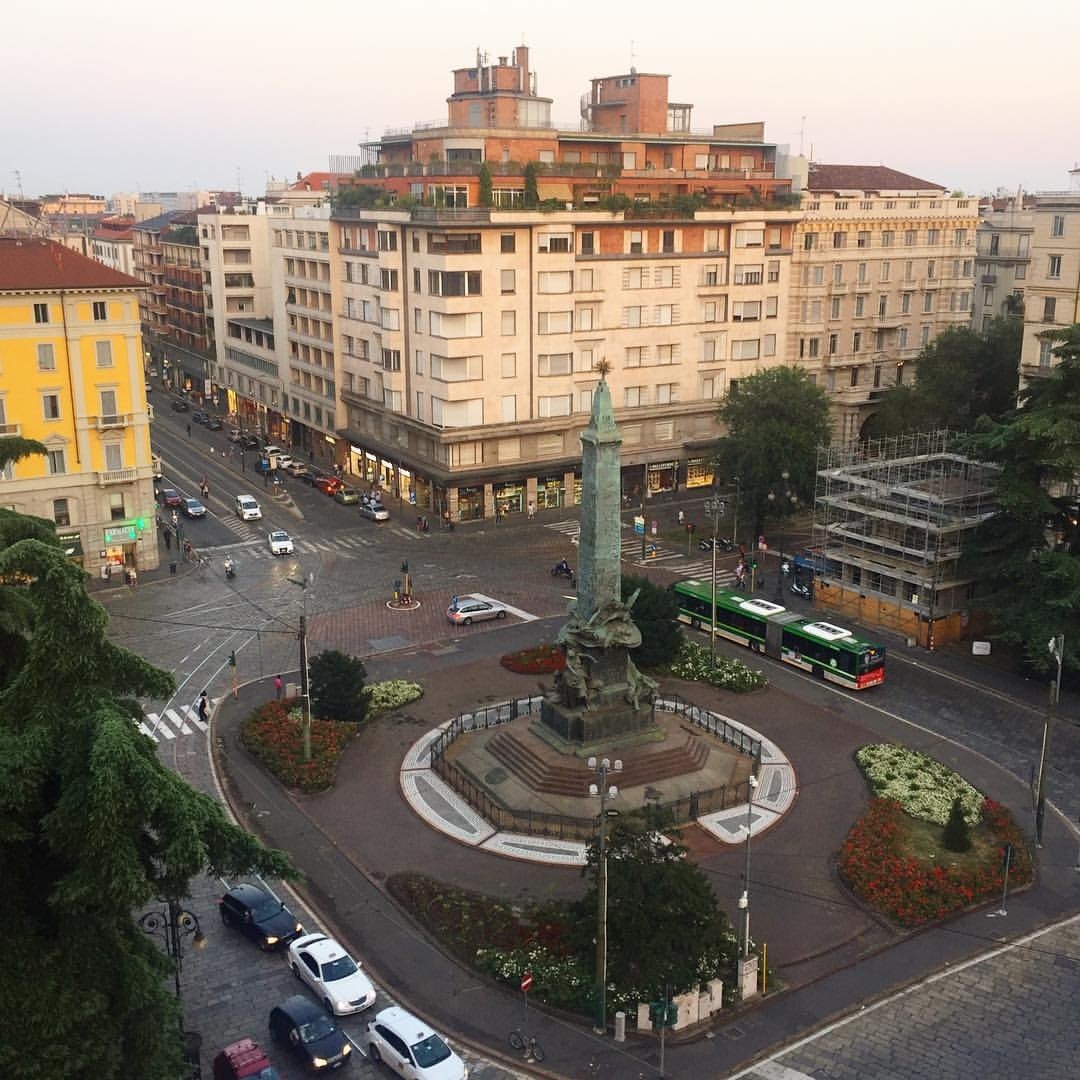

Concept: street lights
[[769, 472, 797, 603], [587, 756, 622, 1032], [704, 499, 725, 667], [139, 895, 208, 1031]]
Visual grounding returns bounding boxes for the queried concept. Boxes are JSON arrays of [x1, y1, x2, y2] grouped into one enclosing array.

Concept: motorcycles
[[550, 564, 575, 579], [698, 536, 734, 553], [781, 557, 812, 600], [225, 563, 233, 579]]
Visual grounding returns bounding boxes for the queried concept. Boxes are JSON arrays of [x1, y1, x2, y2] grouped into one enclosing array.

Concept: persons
[[187, 424, 192, 439], [198, 692, 209, 721], [162, 526, 171, 550], [224, 555, 234, 571], [496, 500, 537, 521], [732, 532, 768, 592], [200, 479, 208, 498], [273, 675, 282, 696]]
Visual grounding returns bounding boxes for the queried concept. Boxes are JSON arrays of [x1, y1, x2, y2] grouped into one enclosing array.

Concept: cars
[[447, 599, 507, 625], [213, 883, 376, 1080], [366, 1006, 469, 1080], [145, 368, 391, 555]]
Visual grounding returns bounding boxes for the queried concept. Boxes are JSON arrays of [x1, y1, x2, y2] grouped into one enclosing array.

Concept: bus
[[667, 579, 886, 690]]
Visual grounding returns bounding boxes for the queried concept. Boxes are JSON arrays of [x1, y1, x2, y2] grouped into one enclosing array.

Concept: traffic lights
[[686, 524, 697, 535], [400, 565, 407, 573], [752, 559, 757, 568], [229, 656, 235, 666], [648, 1002, 678, 1030]]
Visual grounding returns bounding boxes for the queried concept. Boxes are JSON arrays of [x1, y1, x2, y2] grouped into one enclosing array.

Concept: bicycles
[[509, 1028, 545, 1062]]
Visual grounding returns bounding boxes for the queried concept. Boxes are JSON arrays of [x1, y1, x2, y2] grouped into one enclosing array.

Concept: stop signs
[[522, 974, 533, 992]]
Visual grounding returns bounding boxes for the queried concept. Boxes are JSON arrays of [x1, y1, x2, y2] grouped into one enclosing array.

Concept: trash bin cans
[[614, 1012, 625, 1042], [285, 683, 297, 698], [170, 563, 176, 577]]
